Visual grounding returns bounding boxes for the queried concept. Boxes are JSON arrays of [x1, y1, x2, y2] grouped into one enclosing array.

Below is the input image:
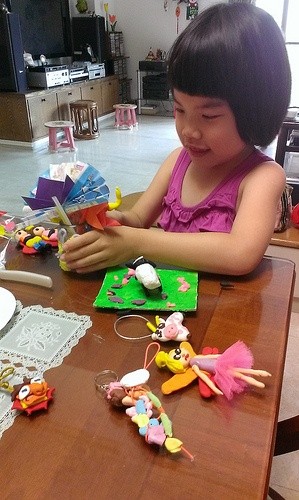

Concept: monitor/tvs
[[16, 0, 74, 61]]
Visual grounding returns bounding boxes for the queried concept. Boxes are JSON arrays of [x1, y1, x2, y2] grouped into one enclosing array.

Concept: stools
[[69, 100, 99, 140], [44, 121, 75, 153], [113, 104, 137, 129]]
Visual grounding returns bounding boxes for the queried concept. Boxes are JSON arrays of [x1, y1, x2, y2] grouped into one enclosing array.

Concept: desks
[[275, 122, 299, 168], [0, 213, 295, 500]]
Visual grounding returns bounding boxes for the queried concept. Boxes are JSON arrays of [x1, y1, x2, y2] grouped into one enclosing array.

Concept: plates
[[0, 286, 16, 331]]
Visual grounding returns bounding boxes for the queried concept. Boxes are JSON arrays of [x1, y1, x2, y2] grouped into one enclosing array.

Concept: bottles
[[289, 129, 299, 148], [58, 219, 87, 273]]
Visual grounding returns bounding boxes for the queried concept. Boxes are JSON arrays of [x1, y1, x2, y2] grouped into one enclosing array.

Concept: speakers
[[72, 16, 107, 77], [0, 13, 28, 93]]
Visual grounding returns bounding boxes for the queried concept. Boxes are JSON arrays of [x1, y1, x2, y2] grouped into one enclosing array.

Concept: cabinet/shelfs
[[0, 74, 120, 143], [109, 31, 133, 105], [136, 70, 172, 114]]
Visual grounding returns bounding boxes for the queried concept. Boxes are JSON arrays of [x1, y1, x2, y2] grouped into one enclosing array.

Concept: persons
[[60, 4, 292, 276], [31, 226, 58, 241], [155, 340, 271, 401], [14, 229, 51, 251]]
